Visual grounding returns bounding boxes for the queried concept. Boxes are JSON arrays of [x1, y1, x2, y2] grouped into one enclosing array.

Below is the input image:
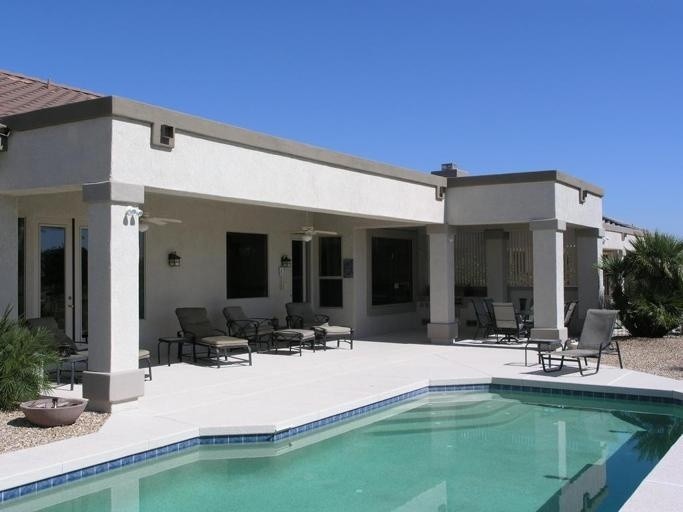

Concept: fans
[[139, 212, 182, 232], [288, 213, 337, 242]]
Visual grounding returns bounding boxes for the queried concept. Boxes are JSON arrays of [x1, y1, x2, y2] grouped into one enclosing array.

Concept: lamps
[[302, 234, 313, 241], [281, 255, 291, 267], [139, 222, 150, 232], [168, 251, 182, 266]]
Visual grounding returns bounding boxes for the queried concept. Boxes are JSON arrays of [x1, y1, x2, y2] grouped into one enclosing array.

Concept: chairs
[[175, 301, 354, 367], [539, 308, 623, 376], [25, 317, 152, 391], [470, 296, 579, 344]]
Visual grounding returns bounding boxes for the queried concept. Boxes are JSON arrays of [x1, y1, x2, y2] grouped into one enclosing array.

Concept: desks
[[525, 340, 563, 367], [158, 338, 194, 367]]
